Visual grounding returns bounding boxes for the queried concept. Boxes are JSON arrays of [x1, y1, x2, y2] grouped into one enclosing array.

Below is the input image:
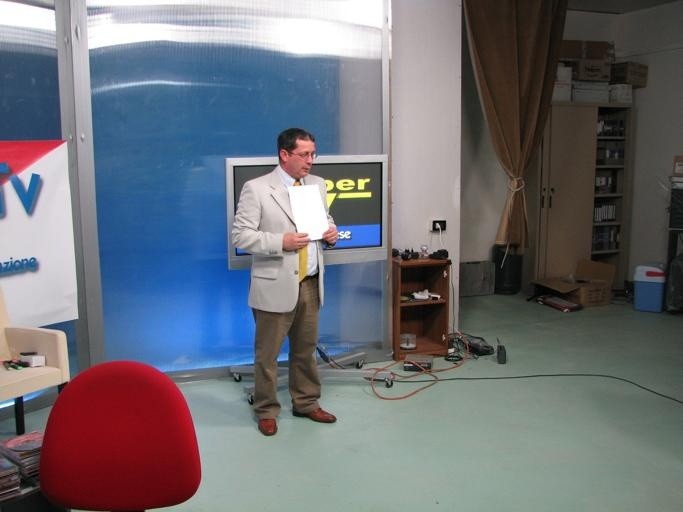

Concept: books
[[0, 431, 46, 500], [594, 203, 616, 222]]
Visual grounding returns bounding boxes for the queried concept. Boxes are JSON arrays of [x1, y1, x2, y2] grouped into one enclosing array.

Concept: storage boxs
[[531, 258, 618, 306], [670, 155, 683, 176], [552, 39, 649, 102], [632, 263, 666, 313]]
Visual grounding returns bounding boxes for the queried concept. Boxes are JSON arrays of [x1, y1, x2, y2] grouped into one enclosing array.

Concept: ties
[[293, 181, 309, 283]]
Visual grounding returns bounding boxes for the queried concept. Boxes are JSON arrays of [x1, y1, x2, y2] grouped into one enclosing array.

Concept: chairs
[[39, 360, 202, 512]]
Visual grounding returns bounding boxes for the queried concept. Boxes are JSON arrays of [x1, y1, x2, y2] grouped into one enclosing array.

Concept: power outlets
[[430, 221, 446, 231]]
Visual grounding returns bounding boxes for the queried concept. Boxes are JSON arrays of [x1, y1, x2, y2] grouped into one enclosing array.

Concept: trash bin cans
[[635, 261, 664, 313]]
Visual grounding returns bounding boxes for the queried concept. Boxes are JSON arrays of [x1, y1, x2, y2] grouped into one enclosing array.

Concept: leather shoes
[[291, 405, 337, 424], [255, 415, 278, 437]]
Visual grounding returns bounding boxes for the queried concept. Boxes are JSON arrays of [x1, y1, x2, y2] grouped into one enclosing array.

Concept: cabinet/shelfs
[[392, 252, 452, 361], [0, 323, 69, 512], [663, 176, 683, 315], [522, 103, 636, 292]]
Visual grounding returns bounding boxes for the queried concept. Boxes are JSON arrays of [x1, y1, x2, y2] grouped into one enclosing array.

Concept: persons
[[230, 128, 340, 436]]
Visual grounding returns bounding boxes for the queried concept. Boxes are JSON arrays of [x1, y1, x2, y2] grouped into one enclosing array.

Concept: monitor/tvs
[[225, 154, 388, 271]]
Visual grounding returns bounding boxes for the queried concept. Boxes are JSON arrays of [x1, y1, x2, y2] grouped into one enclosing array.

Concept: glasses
[[287, 149, 318, 159]]
